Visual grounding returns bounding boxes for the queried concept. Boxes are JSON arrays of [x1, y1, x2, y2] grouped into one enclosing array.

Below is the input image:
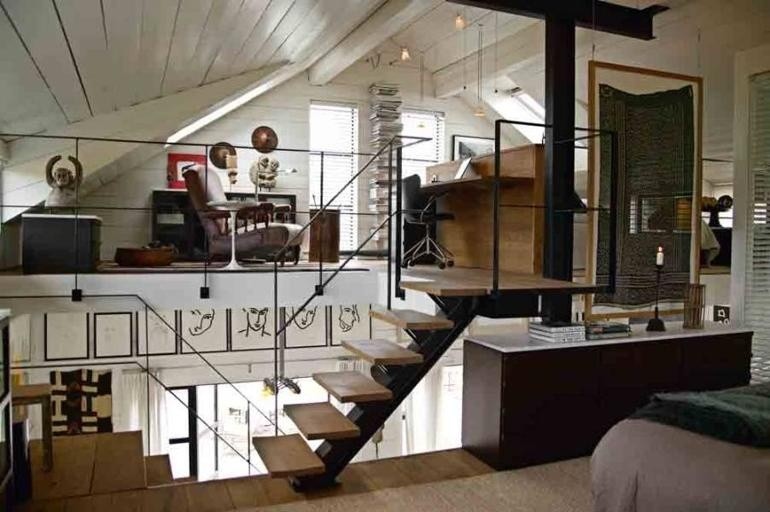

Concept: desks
[[420, 143, 545, 274]]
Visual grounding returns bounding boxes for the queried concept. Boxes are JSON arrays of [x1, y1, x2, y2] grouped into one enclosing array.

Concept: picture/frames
[[167, 152, 207, 189], [452, 135, 495, 162]]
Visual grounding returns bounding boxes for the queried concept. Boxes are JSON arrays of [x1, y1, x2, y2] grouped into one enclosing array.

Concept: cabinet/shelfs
[[152, 188, 297, 261], [22, 213, 101, 272], [1, 309, 14, 490], [461, 321, 754, 470]]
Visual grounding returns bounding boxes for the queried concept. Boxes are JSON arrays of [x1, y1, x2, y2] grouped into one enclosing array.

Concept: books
[[528, 321, 586, 343], [586, 323, 631, 340], [368, 81, 402, 245]]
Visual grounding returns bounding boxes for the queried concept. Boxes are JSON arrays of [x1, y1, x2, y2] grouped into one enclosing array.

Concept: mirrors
[[583, 60, 704, 321]]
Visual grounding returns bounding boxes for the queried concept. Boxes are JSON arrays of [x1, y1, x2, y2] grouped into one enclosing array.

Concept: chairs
[[402, 174, 456, 269], [183, 165, 304, 266]]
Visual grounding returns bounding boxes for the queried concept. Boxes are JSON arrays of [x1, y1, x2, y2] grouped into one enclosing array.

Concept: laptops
[[429, 155, 473, 183]]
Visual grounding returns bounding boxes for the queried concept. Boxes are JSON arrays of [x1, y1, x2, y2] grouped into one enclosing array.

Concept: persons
[[250, 158, 281, 186]]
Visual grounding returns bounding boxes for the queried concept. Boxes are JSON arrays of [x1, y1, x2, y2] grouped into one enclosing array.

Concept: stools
[[12, 383, 54, 470]]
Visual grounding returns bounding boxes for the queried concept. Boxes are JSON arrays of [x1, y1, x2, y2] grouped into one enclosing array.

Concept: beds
[[588, 378, 769, 512]]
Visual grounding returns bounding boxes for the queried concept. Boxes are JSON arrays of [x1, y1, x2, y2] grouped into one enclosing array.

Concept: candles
[[654, 244, 664, 264]]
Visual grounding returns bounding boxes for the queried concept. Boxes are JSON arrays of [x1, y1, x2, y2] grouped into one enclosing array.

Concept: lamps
[[473, 24, 487, 116]]
